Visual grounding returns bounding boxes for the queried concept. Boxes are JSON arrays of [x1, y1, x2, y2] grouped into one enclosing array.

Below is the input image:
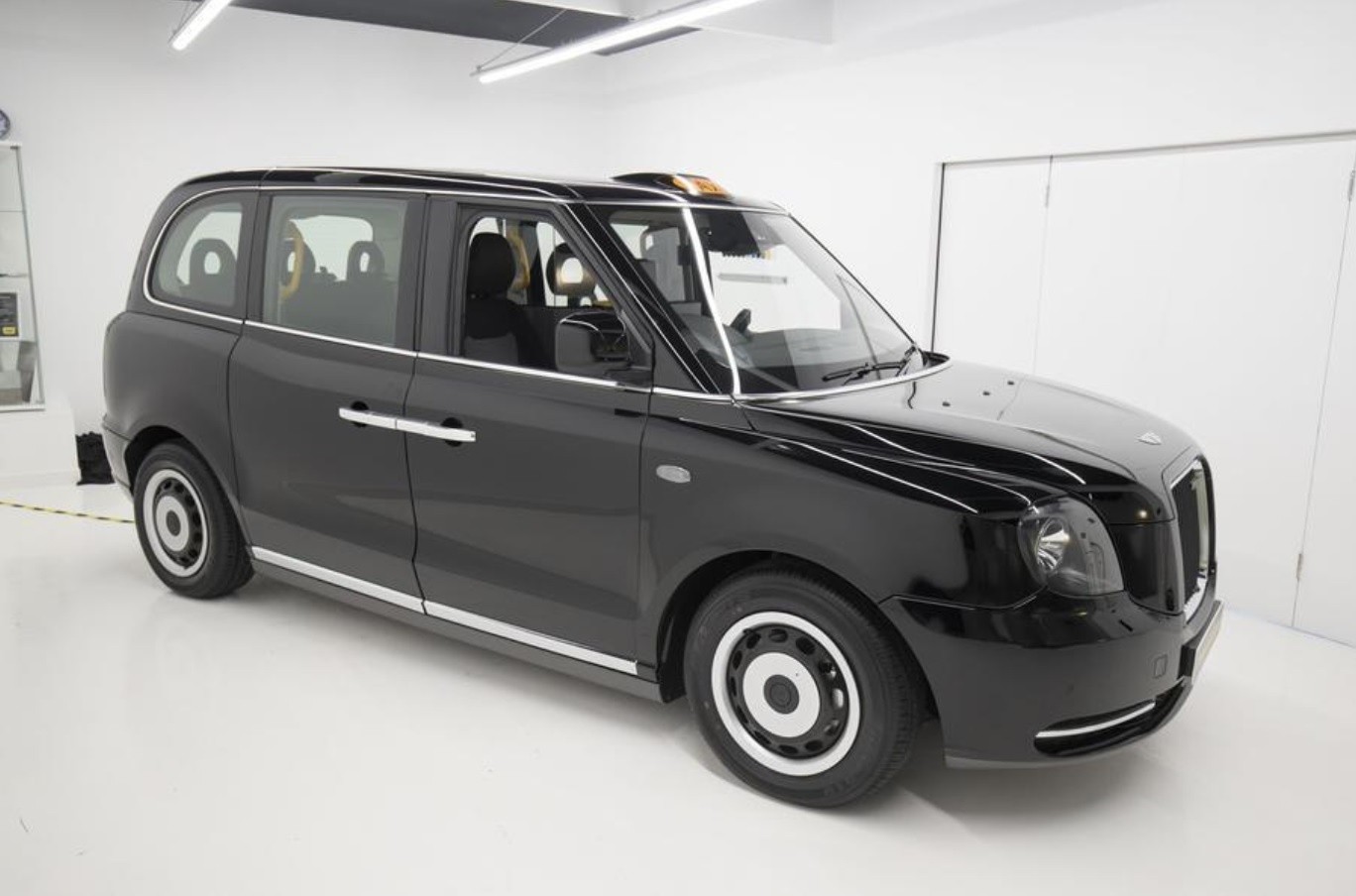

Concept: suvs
[[99, 167, 1221, 815]]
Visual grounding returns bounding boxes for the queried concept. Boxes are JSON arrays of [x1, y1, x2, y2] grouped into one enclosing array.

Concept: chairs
[[179, 238, 236, 305], [284, 240, 337, 333], [460, 232, 544, 368], [338, 241, 395, 339], [547, 244, 613, 336]]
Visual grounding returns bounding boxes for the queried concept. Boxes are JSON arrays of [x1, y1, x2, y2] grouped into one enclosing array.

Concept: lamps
[[470, 0, 757, 85], [166, 0, 230, 50]]
